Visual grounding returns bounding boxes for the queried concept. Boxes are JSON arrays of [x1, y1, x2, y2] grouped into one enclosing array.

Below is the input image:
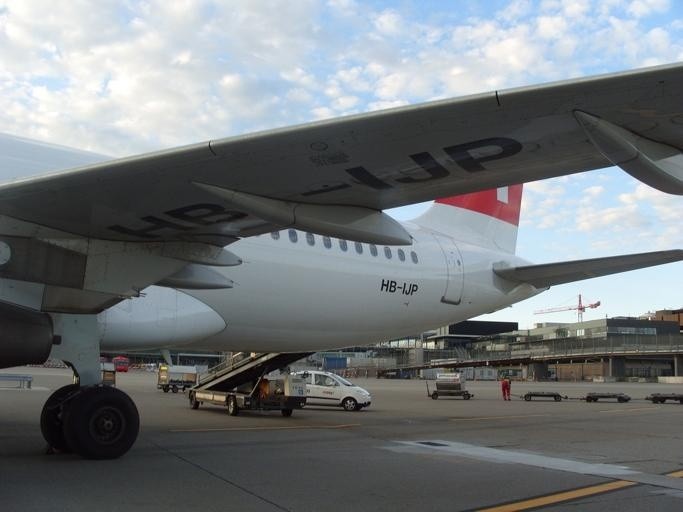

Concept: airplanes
[[0, 64, 678, 461]]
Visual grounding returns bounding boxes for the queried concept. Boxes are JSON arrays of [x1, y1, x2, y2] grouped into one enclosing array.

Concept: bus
[[112, 357, 128, 373]]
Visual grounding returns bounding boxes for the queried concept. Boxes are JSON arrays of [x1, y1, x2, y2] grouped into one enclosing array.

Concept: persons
[[317, 364, 382, 378], [499, 371, 511, 401]]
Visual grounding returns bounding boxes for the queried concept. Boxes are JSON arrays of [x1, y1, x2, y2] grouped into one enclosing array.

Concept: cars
[[293, 370, 371, 411]]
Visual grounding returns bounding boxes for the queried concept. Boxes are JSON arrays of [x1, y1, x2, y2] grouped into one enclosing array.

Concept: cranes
[[533, 295, 601, 324]]
